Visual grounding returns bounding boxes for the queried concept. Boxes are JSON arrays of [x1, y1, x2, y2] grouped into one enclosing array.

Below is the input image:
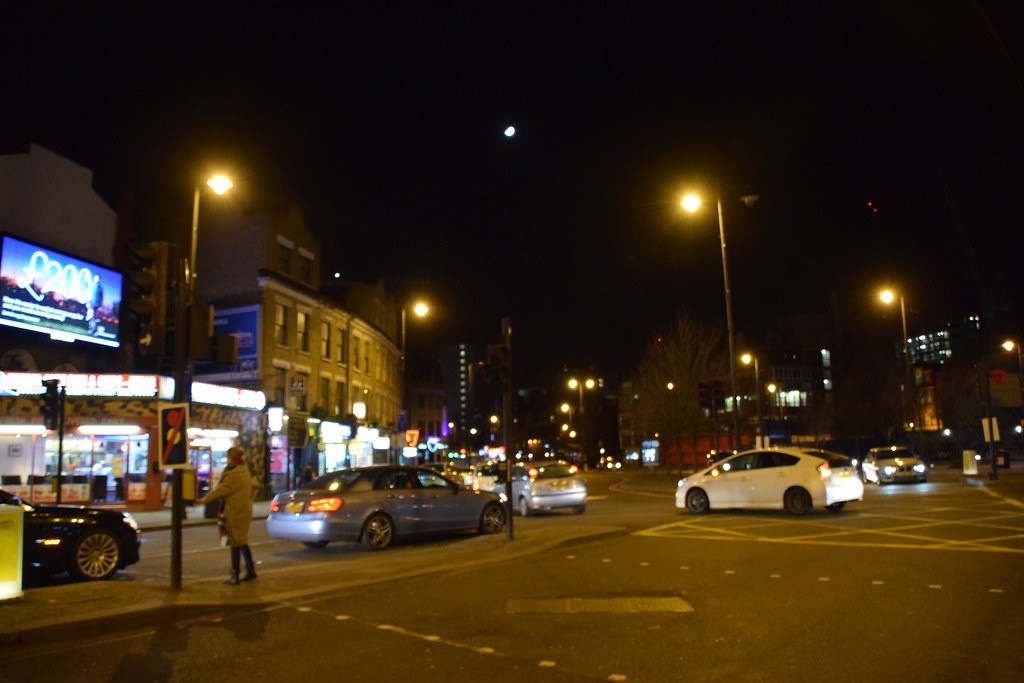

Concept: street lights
[[677, 188, 739, 454], [876, 287, 914, 447], [560, 403, 573, 430], [169, 168, 239, 590], [739, 353, 765, 450], [767, 383, 783, 420], [568, 378, 597, 459], [1001, 338, 1024, 409], [401, 299, 432, 381]]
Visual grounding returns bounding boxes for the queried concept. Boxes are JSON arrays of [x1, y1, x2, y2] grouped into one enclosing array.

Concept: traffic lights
[[40, 380, 58, 430], [119, 241, 165, 361], [488, 345, 510, 381]]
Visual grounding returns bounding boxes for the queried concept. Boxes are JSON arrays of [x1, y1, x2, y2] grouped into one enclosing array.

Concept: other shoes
[[242, 571, 258, 582], [223, 578, 240, 585]]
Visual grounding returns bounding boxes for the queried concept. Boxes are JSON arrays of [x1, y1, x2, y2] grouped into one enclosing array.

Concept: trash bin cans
[[205, 499, 219, 518], [995, 450, 1009, 468]]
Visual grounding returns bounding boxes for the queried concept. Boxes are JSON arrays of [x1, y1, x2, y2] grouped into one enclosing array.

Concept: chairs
[[395, 475, 412, 489]]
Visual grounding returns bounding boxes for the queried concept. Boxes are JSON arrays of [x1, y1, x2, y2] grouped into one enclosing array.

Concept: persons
[[196, 447, 258, 586], [303, 463, 317, 482]]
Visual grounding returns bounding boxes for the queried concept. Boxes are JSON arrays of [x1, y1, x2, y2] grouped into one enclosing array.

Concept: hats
[[227, 446, 244, 461]]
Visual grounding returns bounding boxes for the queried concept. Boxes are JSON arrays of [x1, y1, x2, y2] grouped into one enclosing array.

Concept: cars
[[265, 464, 506, 554], [0, 488, 143, 582], [605, 460, 622, 471], [862, 448, 928, 486], [676, 447, 865, 517], [462, 458, 588, 517]]
